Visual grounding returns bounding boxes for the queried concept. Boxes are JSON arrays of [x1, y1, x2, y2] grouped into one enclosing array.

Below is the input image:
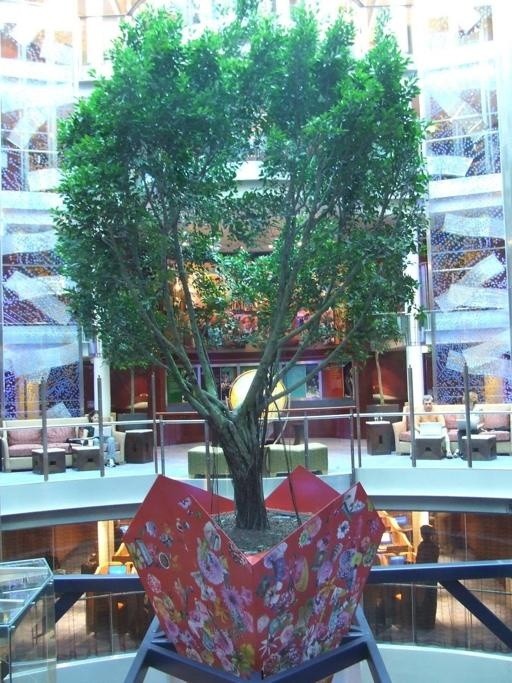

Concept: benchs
[[188, 441, 328, 479]]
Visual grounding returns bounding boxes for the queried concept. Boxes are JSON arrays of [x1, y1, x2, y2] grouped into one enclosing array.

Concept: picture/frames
[[163, 360, 323, 407]]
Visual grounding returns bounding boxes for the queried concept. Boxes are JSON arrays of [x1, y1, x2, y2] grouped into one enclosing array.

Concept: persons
[[80, 411, 117, 468], [453, 392, 483, 458], [415, 524, 439, 625], [410, 396, 452, 460], [114, 520, 126, 551]]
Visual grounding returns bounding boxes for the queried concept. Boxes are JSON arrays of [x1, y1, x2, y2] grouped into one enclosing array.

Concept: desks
[[31, 448, 66, 475], [71, 445, 100, 472], [126, 428, 153, 464], [414, 434, 445, 460], [461, 433, 497, 461], [365, 420, 393, 455]]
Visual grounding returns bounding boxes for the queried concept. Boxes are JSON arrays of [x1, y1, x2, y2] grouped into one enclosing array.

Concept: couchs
[[391, 403, 512, 457], [1, 417, 125, 473]]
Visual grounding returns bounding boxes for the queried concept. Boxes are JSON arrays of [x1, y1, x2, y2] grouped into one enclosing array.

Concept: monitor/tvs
[[108, 563, 132, 575], [119, 523, 130, 536]]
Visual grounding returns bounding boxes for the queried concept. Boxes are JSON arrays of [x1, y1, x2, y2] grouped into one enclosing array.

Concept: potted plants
[[48, 2, 441, 683]]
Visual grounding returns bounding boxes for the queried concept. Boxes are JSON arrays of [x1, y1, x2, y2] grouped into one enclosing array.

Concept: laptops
[[91, 426, 112, 439]]
